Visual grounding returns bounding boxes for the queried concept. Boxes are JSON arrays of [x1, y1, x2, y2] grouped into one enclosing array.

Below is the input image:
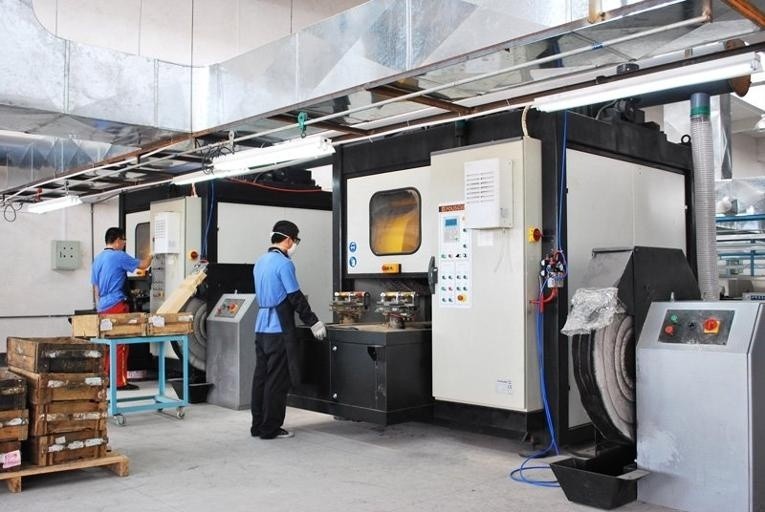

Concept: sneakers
[[276, 427, 295, 438], [118, 382, 140, 390]]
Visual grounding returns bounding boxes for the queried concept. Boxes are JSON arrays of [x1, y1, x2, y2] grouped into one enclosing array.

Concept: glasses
[[294, 236, 300, 245]]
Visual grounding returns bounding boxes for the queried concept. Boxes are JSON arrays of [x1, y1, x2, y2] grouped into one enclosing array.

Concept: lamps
[[173, 135, 338, 186], [532, 52, 764, 113], [28, 195, 82, 214]]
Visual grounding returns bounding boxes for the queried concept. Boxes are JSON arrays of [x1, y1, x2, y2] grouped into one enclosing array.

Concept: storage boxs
[[71, 312, 148, 337], [0, 336, 108, 473], [148, 312, 192, 336]]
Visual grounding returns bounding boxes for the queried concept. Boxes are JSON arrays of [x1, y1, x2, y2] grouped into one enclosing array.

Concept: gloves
[[310, 320, 327, 341]]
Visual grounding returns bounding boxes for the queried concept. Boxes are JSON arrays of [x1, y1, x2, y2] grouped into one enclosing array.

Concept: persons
[[250, 220, 327, 441], [90, 226, 153, 391]]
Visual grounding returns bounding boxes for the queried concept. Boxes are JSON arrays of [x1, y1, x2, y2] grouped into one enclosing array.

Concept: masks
[[285, 238, 298, 257]]
